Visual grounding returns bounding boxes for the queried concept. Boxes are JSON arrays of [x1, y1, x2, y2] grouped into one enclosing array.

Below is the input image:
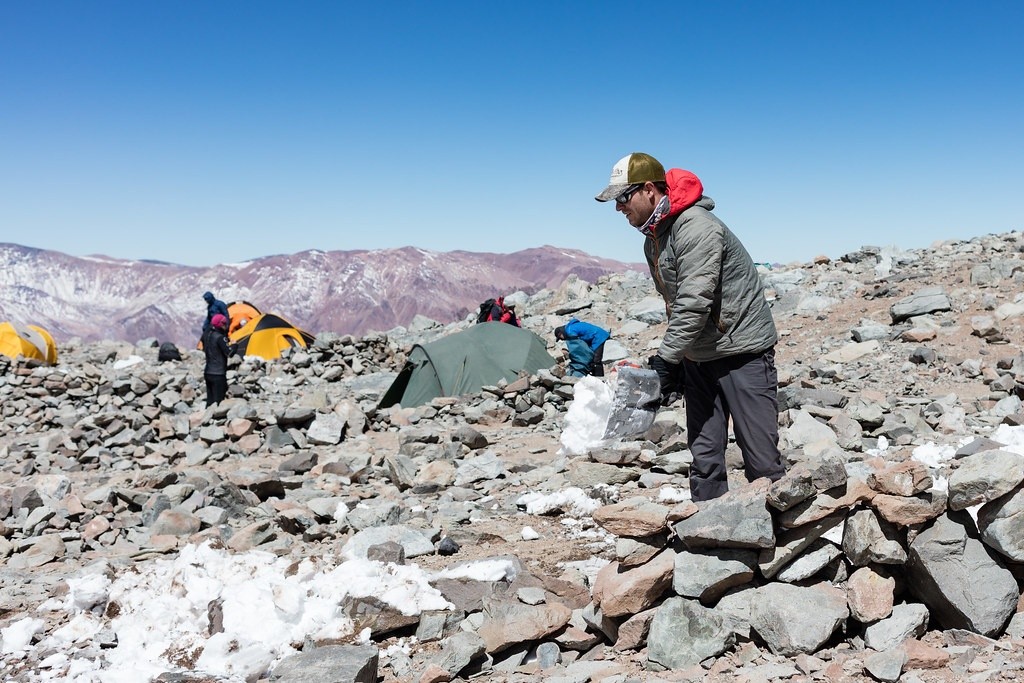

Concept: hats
[[555, 327, 562, 342], [210, 314, 226, 327], [595, 152, 667, 202]]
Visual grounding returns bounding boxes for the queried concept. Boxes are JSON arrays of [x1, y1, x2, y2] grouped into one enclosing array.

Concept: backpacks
[[158, 342, 182, 362], [476, 299, 496, 323]]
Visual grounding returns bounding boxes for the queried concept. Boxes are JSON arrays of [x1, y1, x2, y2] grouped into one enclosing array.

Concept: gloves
[[647, 355, 684, 406]]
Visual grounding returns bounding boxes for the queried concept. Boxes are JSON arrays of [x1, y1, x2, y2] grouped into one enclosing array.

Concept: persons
[[478, 295, 521, 327], [555, 318, 608, 377], [203, 291, 229, 337], [597, 152, 786, 502], [204, 314, 238, 408]]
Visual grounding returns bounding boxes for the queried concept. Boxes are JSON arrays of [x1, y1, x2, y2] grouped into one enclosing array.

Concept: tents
[[376, 321, 557, 410], [0, 322, 57, 363], [196, 300, 260, 350], [227, 313, 317, 361]]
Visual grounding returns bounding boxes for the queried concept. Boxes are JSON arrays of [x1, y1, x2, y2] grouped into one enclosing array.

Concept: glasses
[[615, 184, 645, 204]]
